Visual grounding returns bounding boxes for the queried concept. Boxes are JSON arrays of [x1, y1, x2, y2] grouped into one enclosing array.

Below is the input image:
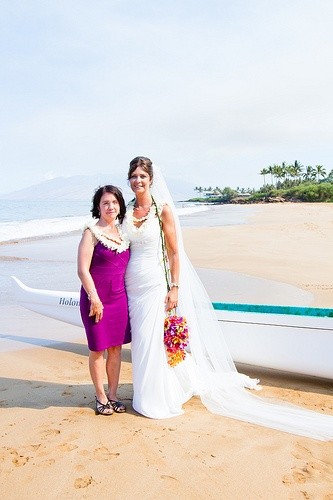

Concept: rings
[[174, 305, 177, 307]]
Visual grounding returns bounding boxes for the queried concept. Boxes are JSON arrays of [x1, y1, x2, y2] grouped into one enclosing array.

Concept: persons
[[115, 156, 333, 442], [77, 185, 131, 415]]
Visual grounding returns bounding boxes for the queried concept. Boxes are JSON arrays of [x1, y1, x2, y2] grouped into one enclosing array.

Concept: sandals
[[94, 395, 127, 415]]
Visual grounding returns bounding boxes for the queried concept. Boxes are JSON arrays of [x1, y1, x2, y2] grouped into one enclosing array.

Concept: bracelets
[[170, 282, 178, 288], [87, 289, 96, 300]]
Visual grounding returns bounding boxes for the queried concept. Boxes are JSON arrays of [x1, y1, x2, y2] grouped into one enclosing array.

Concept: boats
[[10, 275, 333, 383]]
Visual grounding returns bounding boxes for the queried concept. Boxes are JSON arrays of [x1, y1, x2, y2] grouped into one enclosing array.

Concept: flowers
[[80, 224, 131, 253], [163, 307, 191, 366], [126, 197, 160, 222]]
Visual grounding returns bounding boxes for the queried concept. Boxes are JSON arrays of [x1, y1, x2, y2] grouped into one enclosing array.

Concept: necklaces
[[129, 200, 150, 222], [92, 224, 123, 244]]
